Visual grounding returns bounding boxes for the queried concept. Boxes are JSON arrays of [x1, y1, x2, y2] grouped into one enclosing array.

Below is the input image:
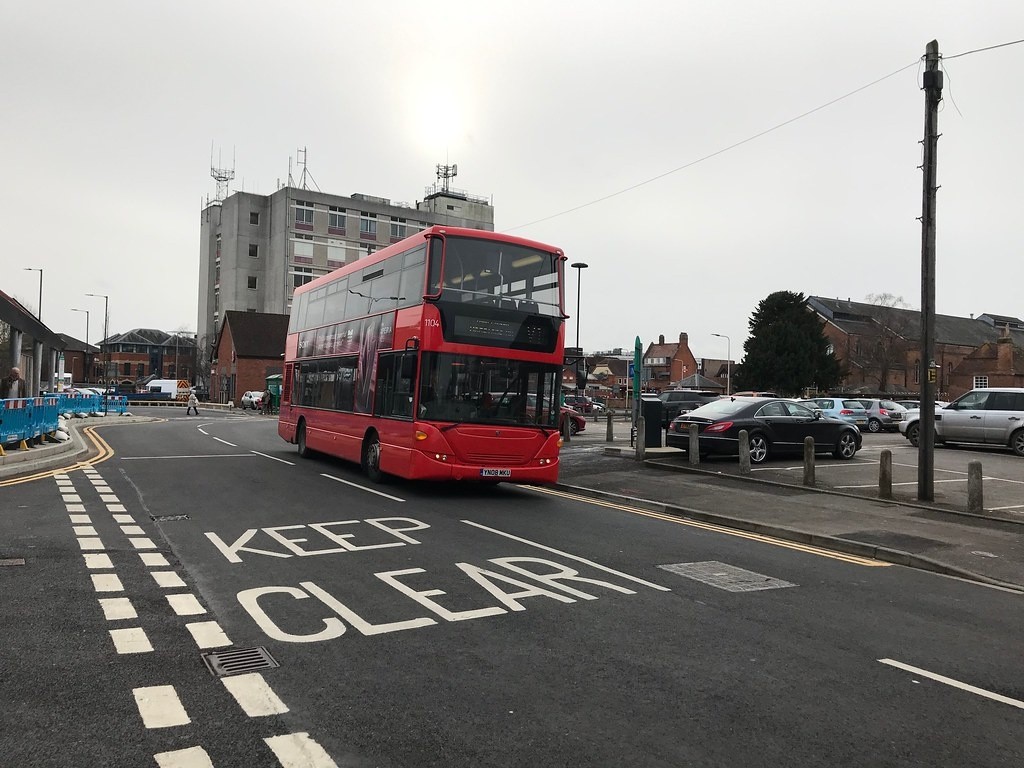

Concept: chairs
[[494, 294, 516, 310], [818, 402, 824, 408], [517, 298, 539, 314], [913, 405, 917, 408]]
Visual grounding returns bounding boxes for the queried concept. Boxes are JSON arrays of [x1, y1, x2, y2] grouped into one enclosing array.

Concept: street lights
[[710, 334, 730, 395], [24, 268, 42, 321], [571, 263, 589, 389], [71, 308, 89, 382], [85, 294, 108, 382], [674, 358, 684, 380]]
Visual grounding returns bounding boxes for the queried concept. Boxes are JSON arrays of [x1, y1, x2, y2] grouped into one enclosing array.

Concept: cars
[[463, 393, 586, 436], [658, 390, 721, 430], [241, 391, 265, 410], [730, 390, 824, 419], [811, 397, 869, 425], [894, 399, 945, 410], [852, 398, 909, 433], [667, 396, 863, 464], [564, 395, 606, 413]]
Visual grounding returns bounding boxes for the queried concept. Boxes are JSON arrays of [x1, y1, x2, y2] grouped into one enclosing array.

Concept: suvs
[[899, 388, 1024, 457]]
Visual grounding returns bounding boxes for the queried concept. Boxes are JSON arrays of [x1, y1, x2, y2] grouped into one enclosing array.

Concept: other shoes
[[187, 414, 190, 415], [196, 413, 200, 415]]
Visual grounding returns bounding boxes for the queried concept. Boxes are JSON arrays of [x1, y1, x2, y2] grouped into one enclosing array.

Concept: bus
[[276, 223, 570, 486]]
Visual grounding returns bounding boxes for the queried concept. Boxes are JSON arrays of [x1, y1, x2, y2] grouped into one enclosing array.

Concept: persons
[[187, 390, 200, 415], [0, 367, 26, 399], [259, 388, 274, 415]]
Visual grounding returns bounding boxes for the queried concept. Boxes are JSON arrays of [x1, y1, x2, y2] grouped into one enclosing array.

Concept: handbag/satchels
[[194, 398, 199, 403], [261, 396, 265, 402]]
